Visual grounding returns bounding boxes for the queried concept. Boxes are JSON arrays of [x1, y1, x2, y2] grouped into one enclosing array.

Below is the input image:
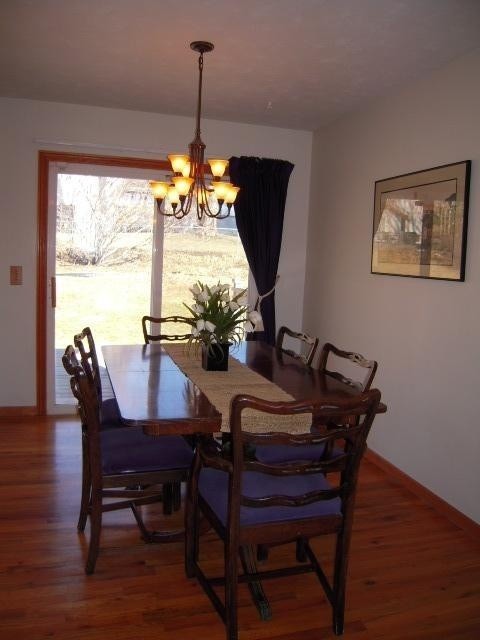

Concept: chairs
[[248, 324, 378, 566], [185, 388, 382, 640], [139, 316, 200, 343], [60, 327, 193, 575]]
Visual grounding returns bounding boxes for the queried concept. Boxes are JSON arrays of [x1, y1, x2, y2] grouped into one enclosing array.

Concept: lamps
[[149, 36, 240, 224]]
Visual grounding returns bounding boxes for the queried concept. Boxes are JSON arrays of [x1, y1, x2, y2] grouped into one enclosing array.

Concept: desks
[[101, 340, 389, 580]]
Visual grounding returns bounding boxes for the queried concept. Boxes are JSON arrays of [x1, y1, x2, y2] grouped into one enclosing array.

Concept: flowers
[[178, 278, 260, 362]]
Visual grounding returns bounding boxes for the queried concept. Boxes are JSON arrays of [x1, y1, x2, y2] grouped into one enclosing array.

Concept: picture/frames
[[366, 158, 472, 283]]
